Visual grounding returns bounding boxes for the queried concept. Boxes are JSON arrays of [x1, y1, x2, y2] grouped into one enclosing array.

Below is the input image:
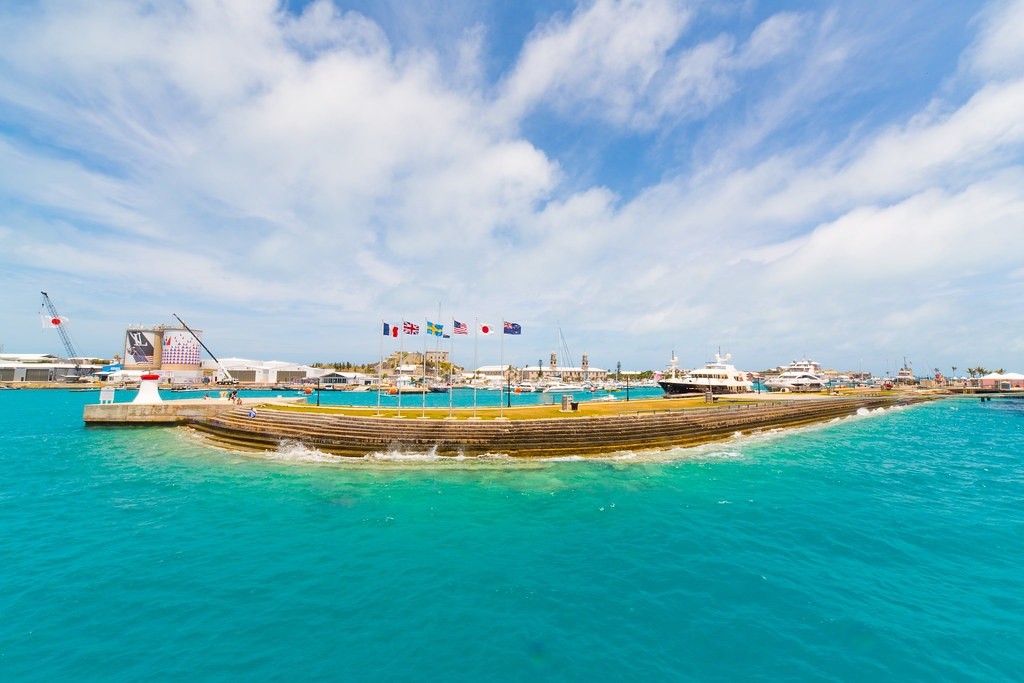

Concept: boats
[[502, 328, 635, 393], [763, 371, 828, 392], [656, 347, 755, 394], [429, 384, 450, 392]]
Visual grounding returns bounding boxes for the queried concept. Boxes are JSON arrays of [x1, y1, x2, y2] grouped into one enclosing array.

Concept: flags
[[42, 316, 68, 328], [504, 321, 521, 335], [426, 320, 442, 336], [453, 320, 468, 335], [383, 323, 399, 338], [403, 320, 419, 335], [476, 322, 494, 336]]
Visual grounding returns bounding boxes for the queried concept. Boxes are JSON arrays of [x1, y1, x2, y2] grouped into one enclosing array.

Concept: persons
[[248, 406, 256, 418], [204, 393, 210, 400], [228, 388, 242, 405]]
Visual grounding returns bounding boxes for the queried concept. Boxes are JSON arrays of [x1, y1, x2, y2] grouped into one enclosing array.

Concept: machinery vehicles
[[38, 290, 96, 377], [172, 312, 240, 385]]
